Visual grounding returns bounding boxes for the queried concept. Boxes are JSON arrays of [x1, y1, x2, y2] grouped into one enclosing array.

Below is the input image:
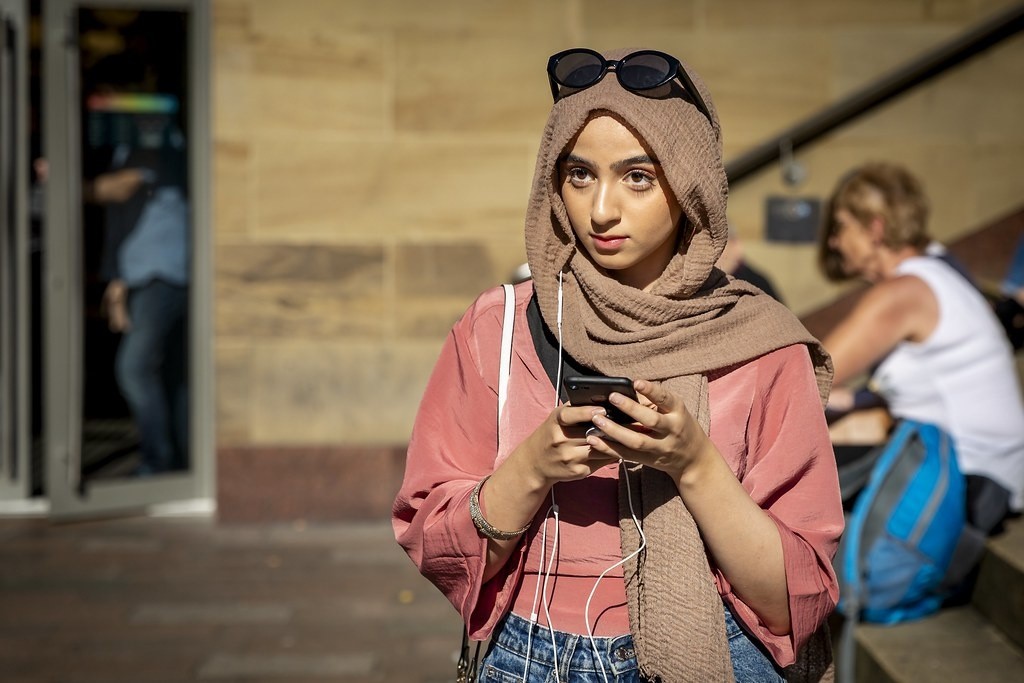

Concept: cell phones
[[564, 376, 641, 427]]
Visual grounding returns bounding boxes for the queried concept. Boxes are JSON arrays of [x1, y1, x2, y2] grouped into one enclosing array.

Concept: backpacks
[[835, 420, 967, 626]]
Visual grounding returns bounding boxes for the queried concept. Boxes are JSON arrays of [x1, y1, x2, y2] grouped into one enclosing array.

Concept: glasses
[[547, 48, 713, 129]]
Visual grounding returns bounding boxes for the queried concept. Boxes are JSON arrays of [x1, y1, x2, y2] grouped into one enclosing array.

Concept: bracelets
[[466, 472, 533, 538]]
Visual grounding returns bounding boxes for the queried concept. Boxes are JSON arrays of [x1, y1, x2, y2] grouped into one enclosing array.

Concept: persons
[[780, 161, 1024, 574], [387, 60, 850, 682], [32, 61, 195, 480]]
